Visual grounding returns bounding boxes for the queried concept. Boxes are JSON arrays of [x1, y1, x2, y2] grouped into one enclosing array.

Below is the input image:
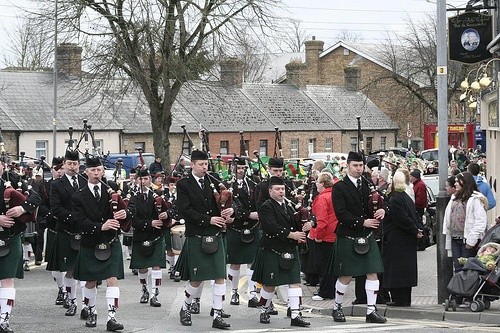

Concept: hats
[[412, 169, 421, 179], [52, 150, 101, 167], [465, 163, 479, 176], [191, 151, 285, 185], [130, 164, 163, 179], [348, 151, 364, 161]]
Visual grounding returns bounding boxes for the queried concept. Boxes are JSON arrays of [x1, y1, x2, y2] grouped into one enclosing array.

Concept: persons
[[0, 148, 500, 333]]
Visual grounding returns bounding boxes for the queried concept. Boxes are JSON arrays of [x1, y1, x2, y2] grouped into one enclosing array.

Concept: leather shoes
[[55, 291, 124, 331], [0, 323, 14, 333], [140, 294, 311, 328], [332, 309, 387, 323]]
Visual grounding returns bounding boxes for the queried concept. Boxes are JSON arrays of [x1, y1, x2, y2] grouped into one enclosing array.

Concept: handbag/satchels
[[201, 235, 220, 254], [140, 240, 154, 258], [241, 229, 255, 244], [0, 238, 11, 257], [353, 236, 370, 255], [279, 252, 295, 271], [70, 235, 82, 250], [94, 241, 112, 261]]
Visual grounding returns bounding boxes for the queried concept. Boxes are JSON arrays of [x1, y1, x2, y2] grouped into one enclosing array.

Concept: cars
[[370, 148, 417, 158], [310, 153, 349, 166], [422, 174, 451, 197], [214, 155, 254, 166], [32, 160, 53, 182], [421, 148, 438, 161], [417, 184, 437, 249], [285, 158, 315, 174]]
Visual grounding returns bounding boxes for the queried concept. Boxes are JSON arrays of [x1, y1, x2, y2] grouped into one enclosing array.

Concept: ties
[[94, 186, 100, 200], [71, 176, 78, 190], [199, 179, 204, 188], [144, 193, 148, 200], [357, 179, 361, 190]]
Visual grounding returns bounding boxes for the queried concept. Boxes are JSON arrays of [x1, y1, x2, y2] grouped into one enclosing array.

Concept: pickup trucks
[[107, 153, 154, 178]]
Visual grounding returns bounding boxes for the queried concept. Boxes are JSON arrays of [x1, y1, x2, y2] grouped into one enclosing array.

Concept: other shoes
[[126, 251, 138, 275], [24, 265, 30, 271], [35, 260, 41, 265], [302, 277, 310, 286], [312, 293, 407, 307], [168, 265, 174, 273]]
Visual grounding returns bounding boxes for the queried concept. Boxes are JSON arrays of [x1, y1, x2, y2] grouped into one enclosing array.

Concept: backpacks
[[426, 187, 436, 215]]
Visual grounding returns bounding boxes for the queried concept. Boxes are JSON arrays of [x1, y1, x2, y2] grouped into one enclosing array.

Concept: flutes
[[0, 114, 401, 254]]
